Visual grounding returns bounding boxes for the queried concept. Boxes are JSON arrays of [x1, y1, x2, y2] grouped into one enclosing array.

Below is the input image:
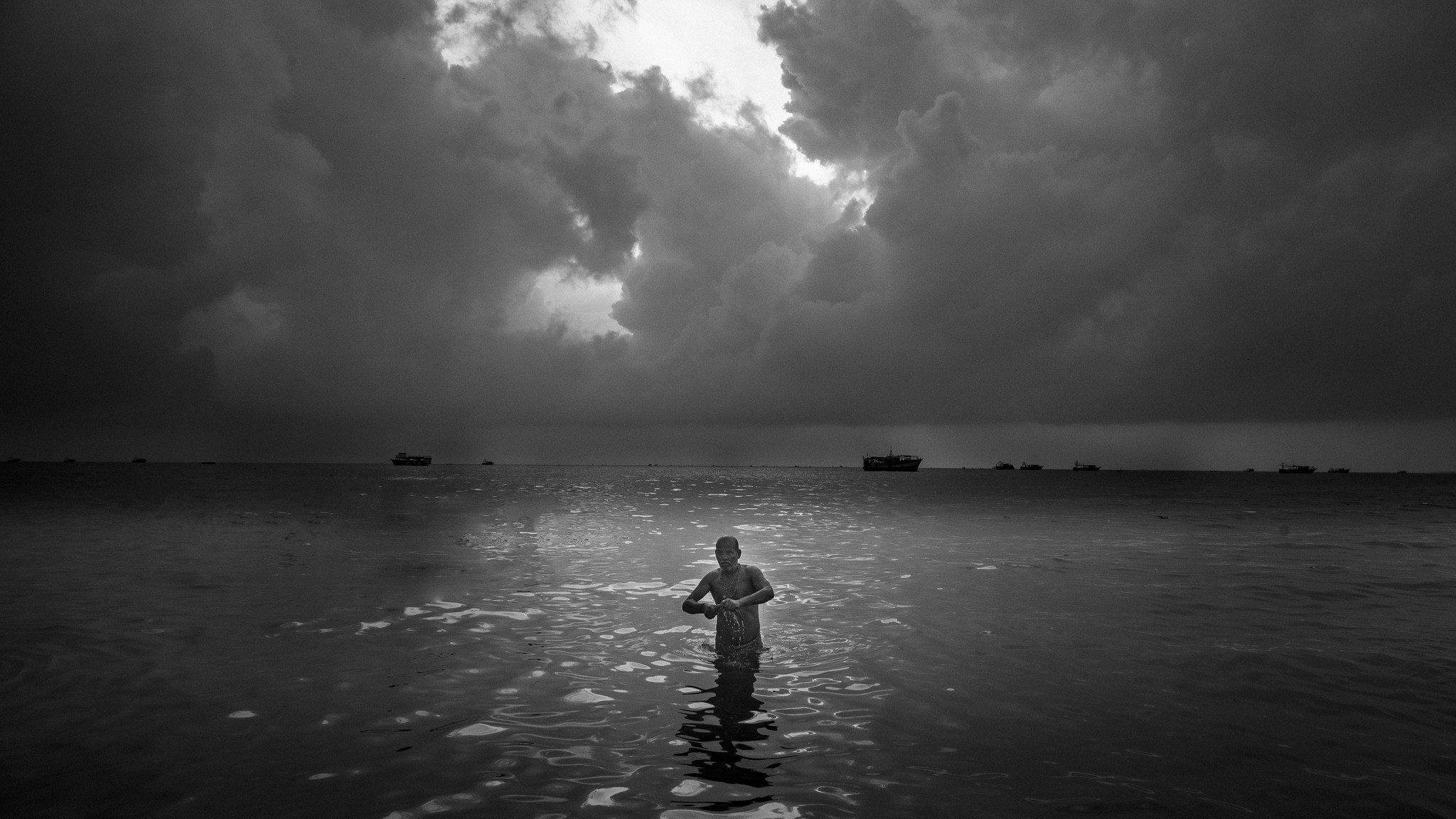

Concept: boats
[[390, 449, 432, 466], [992, 460, 1015, 470], [1397, 470, 1408, 473], [863, 446, 923, 472], [1020, 461, 1044, 470], [1073, 460, 1102, 471], [1328, 467, 1350, 473], [1279, 461, 1317, 474], [482, 459, 495, 465]]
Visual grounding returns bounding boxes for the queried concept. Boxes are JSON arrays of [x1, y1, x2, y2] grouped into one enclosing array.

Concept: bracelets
[[700, 603, 708, 614]]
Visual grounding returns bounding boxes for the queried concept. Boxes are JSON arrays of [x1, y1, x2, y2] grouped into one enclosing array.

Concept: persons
[[682, 536, 774, 657]]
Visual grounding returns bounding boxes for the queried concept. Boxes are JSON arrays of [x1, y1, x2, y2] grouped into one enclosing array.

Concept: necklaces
[[720, 564, 741, 599]]
